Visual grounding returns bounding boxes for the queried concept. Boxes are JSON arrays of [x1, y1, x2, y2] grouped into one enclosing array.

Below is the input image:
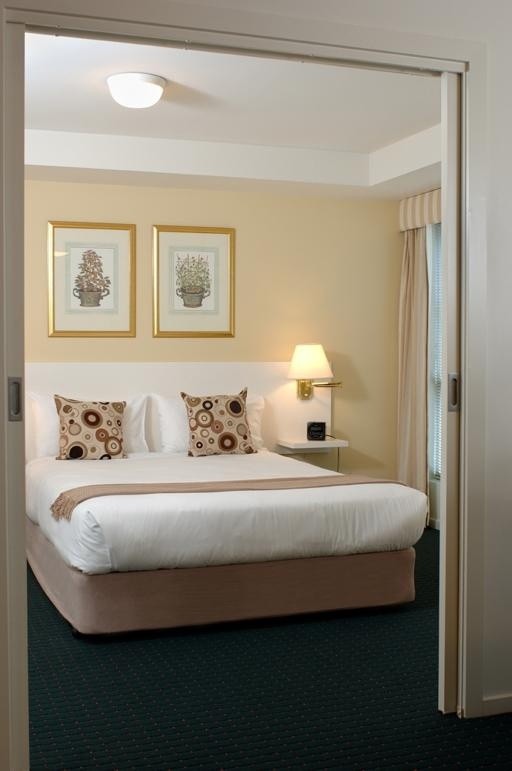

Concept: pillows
[[26, 385, 268, 461]]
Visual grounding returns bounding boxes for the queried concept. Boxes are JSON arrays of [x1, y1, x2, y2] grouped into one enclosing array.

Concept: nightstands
[[273, 428, 348, 474]]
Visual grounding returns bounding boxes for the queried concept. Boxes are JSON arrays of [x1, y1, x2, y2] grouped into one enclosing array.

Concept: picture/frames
[[47, 220, 138, 338], [152, 224, 237, 339]]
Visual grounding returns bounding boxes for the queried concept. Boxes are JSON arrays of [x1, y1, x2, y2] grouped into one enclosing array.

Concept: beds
[[23, 360, 430, 636]]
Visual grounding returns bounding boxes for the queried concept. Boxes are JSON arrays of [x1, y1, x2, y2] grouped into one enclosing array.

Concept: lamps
[[287, 343, 341, 399], [106, 72, 167, 110]]
